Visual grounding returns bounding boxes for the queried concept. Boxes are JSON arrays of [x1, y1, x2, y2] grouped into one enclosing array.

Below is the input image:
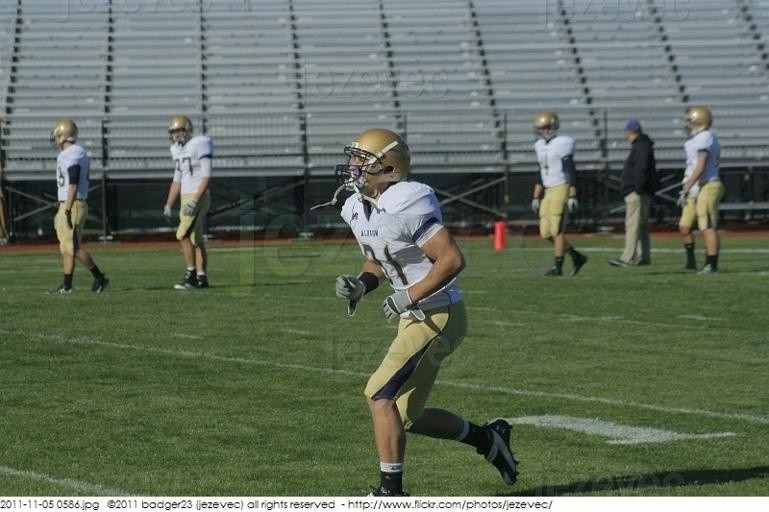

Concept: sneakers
[[92, 278, 108, 292], [184, 276, 209, 288], [50, 287, 72, 296], [696, 264, 718, 275], [608, 259, 630, 267], [367, 485, 410, 496], [174, 269, 195, 289], [482, 419, 520, 486], [569, 255, 587, 276], [544, 269, 561, 276]]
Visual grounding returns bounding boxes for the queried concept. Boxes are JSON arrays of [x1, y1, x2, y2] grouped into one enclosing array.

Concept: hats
[[624, 121, 640, 130]]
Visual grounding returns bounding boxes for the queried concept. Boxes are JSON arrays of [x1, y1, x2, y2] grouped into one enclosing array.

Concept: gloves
[[532, 197, 540, 213], [163, 208, 172, 224], [676, 191, 689, 207], [64, 210, 72, 229], [335, 276, 365, 317], [381, 290, 426, 321], [182, 200, 196, 216], [568, 196, 578, 213]]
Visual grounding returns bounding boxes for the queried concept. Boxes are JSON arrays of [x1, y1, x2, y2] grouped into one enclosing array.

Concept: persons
[[607, 118, 658, 268], [529, 111, 589, 278], [331, 127, 520, 497], [160, 114, 214, 290], [676, 105, 726, 276], [44, 117, 111, 294]]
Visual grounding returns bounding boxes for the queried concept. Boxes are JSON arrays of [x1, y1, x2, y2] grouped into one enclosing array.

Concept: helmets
[[335, 129, 410, 192], [49, 120, 78, 149], [168, 116, 193, 146], [684, 108, 712, 133], [533, 112, 559, 140]]
